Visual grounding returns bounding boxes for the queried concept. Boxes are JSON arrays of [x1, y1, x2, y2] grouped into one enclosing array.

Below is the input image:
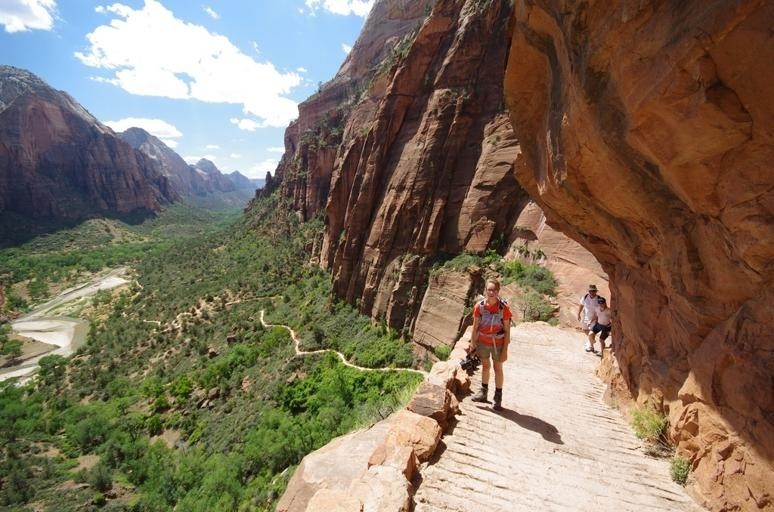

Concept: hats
[[598, 298, 606, 304], [588, 285, 598, 291]]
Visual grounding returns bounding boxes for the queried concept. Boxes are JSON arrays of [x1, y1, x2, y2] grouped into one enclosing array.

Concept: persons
[[576, 284, 602, 353], [585, 297, 612, 355], [470, 279, 513, 410]]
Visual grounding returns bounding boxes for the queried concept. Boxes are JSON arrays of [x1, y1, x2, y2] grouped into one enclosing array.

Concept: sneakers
[[471, 389, 487, 402], [586, 347, 594, 351], [597, 352, 603, 356], [493, 396, 502, 410]]
